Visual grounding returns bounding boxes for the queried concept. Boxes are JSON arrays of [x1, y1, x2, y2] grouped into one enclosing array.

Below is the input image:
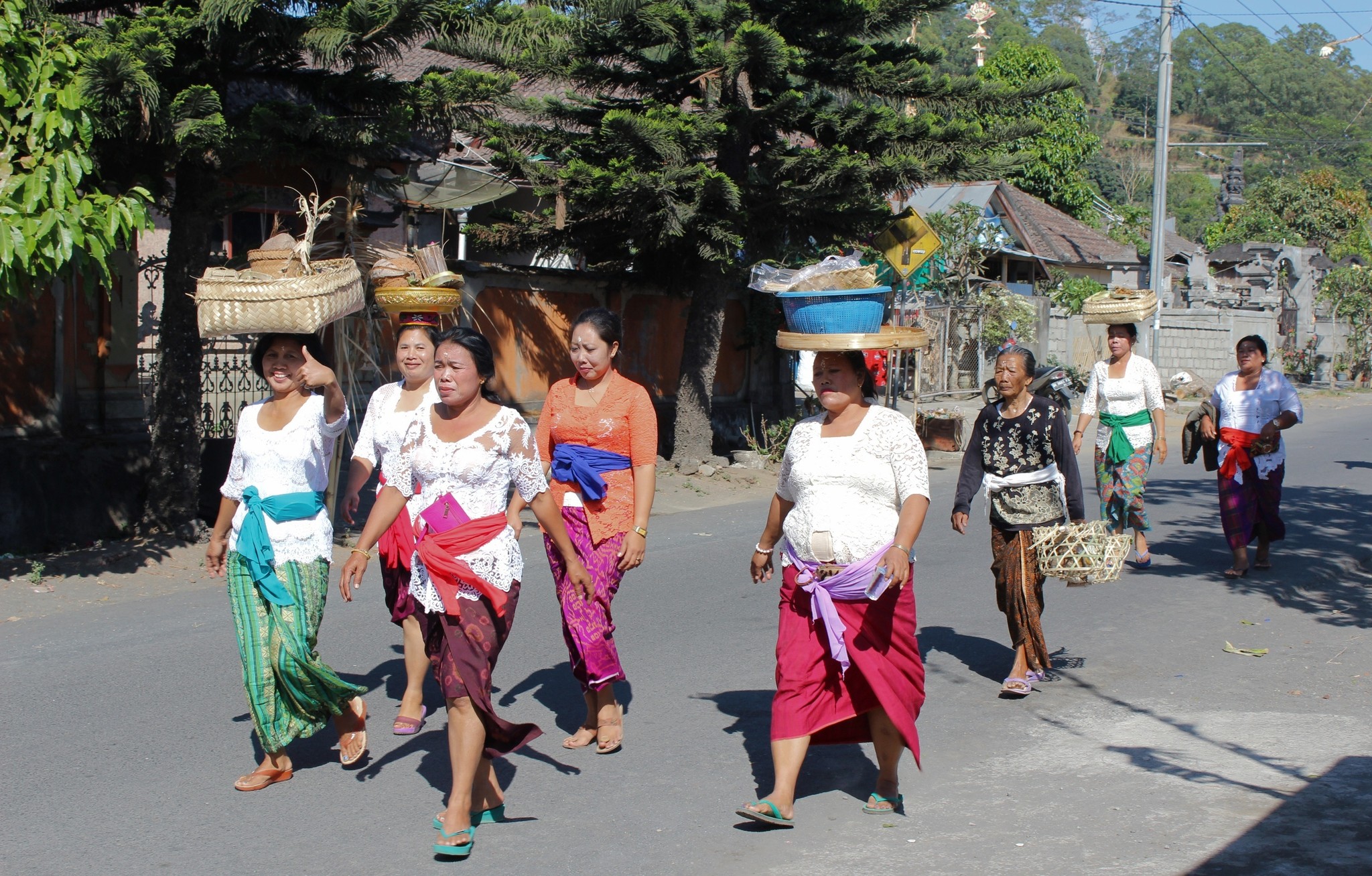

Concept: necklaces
[[1013, 396, 1031, 413], [584, 378, 597, 393]]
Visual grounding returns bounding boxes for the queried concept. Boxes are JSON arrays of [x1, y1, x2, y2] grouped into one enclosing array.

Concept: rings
[[591, 581, 593, 583], [636, 558, 641, 561], [304, 385, 309, 389]]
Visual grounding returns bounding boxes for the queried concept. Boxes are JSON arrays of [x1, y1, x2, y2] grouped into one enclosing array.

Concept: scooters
[[982, 320, 1075, 425]]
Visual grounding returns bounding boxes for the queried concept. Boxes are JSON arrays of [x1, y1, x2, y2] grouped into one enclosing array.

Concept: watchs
[[1271, 418, 1281, 429], [631, 525, 648, 538]]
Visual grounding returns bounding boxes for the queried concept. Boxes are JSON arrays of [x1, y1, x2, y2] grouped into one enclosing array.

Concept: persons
[[736, 350, 931, 826], [206, 334, 368, 792], [948, 345, 1087, 694], [507, 303, 657, 754], [351, 324, 595, 855], [1201, 334, 1304, 578], [1071, 323, 1167, 568], [340, 328, 446, 735]]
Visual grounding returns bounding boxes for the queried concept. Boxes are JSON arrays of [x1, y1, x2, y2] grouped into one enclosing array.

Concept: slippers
[[1000, 678, 1031, 695], [862, 792, 903, 815], [394, 705, 426, 735], [432, 827, 474, 856], [1224, 565, 1249, 578], [1134, 549, 1151, 567], [1254, 560, 1272, 570], [734, 801, 795, 826], [339, 697, 367, 765], [563, 725, 596, 749], [433, 803, 505, 830], [235, 768, 293, 791], [1026, 670, 1046, 682], [596, 704, 623, 753]]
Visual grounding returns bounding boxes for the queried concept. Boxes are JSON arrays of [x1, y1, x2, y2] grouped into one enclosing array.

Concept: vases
[[1300, 373, 1314, 384], [1320, 371, 1330, 381], [1284, 373, 1299, 383], [1336, 372, 1348, 381]]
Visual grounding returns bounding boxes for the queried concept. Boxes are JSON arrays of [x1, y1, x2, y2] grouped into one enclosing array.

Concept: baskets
[[776, 326, 929, 351], [775, 286, 892, 333], [1027, 521, 1111, 579], [185, 254, 365, 338], [1081, 289, 1158, 324], [246, 250, 310, 279], [787, 264, 890, 291], [1068, 534, 1131, 585]]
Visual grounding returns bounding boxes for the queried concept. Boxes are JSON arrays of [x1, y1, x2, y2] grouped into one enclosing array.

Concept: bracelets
[[889, 544, 909, 558], [1073, 430, 1083, 438], [351, 548, 371, 561], [755, 543, 774, 554], [1156, 437, 1166, 441]]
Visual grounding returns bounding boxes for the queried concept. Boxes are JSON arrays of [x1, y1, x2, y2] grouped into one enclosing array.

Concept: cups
[[864, 566, 893, 601]]
